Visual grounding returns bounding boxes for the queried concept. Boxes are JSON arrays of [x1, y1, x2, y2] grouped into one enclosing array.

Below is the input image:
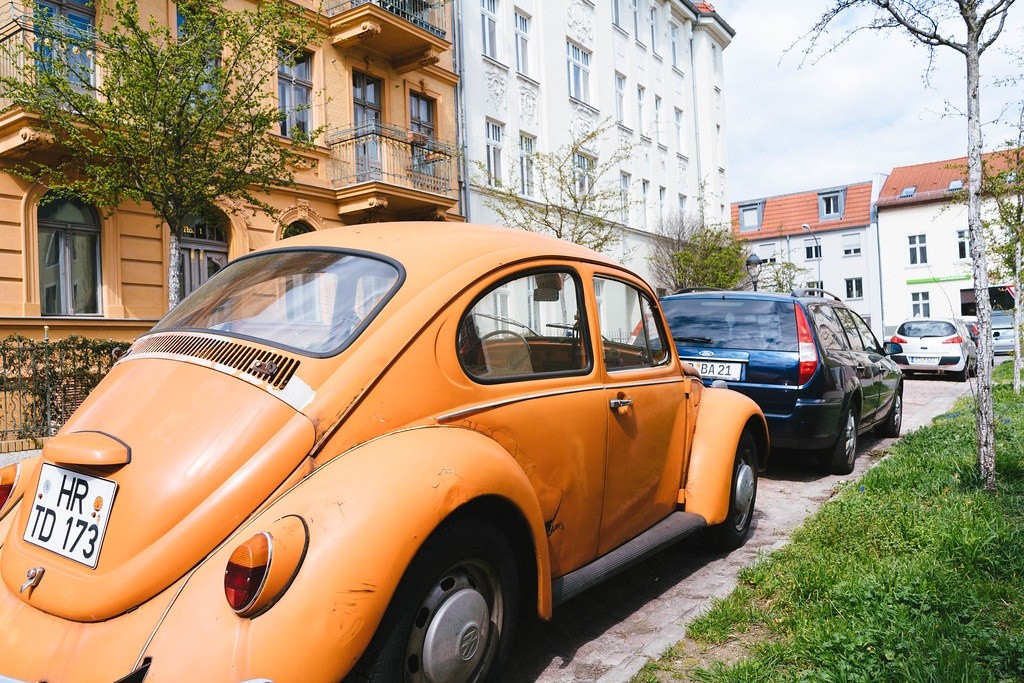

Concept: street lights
[[746, 254, 762, 292], [802, 224, 821, 297]]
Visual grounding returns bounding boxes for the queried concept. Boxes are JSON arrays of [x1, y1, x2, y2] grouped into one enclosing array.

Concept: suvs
[[628, 286, 904, 476]]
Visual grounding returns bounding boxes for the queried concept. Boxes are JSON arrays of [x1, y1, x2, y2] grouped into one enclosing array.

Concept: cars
[[963, 319, 1000, 377], [989, 310, 1015, 353], [1, 221, 771, 683], [890, 317, 977, 382]]
